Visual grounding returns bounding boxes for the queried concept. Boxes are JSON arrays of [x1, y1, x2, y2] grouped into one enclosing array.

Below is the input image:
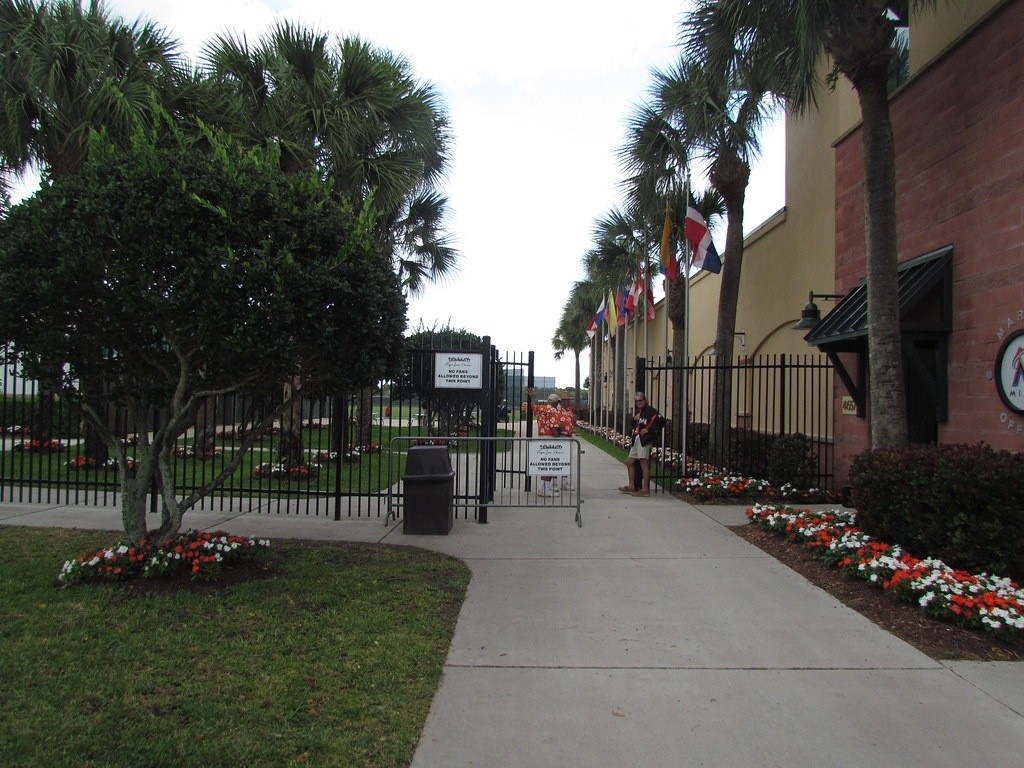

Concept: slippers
[[618, 484, 638, 492], [630, 490, 649, 497]]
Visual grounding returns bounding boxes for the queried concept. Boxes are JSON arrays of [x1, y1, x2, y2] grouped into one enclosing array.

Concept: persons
[[527, 387, 573, 498], [549, 391, 577, 492], [500, 398, 507, 417], [618, 392, 665, 497]]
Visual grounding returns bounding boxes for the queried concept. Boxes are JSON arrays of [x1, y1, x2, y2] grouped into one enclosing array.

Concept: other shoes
[[550, 480, 559, 490], [561, 478, 575, 491], [539, 489, 560, 497]]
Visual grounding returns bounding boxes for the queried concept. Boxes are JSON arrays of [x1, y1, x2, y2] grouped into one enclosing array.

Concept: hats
[[561, 391, 571, 399], [548, 394, 562, 402]]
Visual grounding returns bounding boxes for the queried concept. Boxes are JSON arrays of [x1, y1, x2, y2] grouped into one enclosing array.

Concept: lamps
[[708, 336, 744, 356], [583, 377, 590, 390], [666, 349, 672, 367], [791, 291, 845, 331]]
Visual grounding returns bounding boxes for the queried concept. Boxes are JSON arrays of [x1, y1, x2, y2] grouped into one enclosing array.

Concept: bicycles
[[372, 412, 385, 426]]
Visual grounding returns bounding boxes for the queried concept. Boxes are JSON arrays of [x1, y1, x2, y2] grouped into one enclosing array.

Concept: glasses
[[635, 399, 643, 402]]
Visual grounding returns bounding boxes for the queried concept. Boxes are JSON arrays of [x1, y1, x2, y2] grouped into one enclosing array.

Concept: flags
[[603, 294, 610, 327], [685, 180, 722, 274], [659, 202, 677, 280], [586, 293, 604, 339], [615, 284, 631, 327], [621, 272, 634, 318], [625, 270, 644, 316], [642, 251, 655, 321], [609, 290, 618, 338]]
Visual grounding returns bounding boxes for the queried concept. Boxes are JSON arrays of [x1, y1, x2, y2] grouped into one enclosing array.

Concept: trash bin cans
[[401, 445, 455, 536]]
